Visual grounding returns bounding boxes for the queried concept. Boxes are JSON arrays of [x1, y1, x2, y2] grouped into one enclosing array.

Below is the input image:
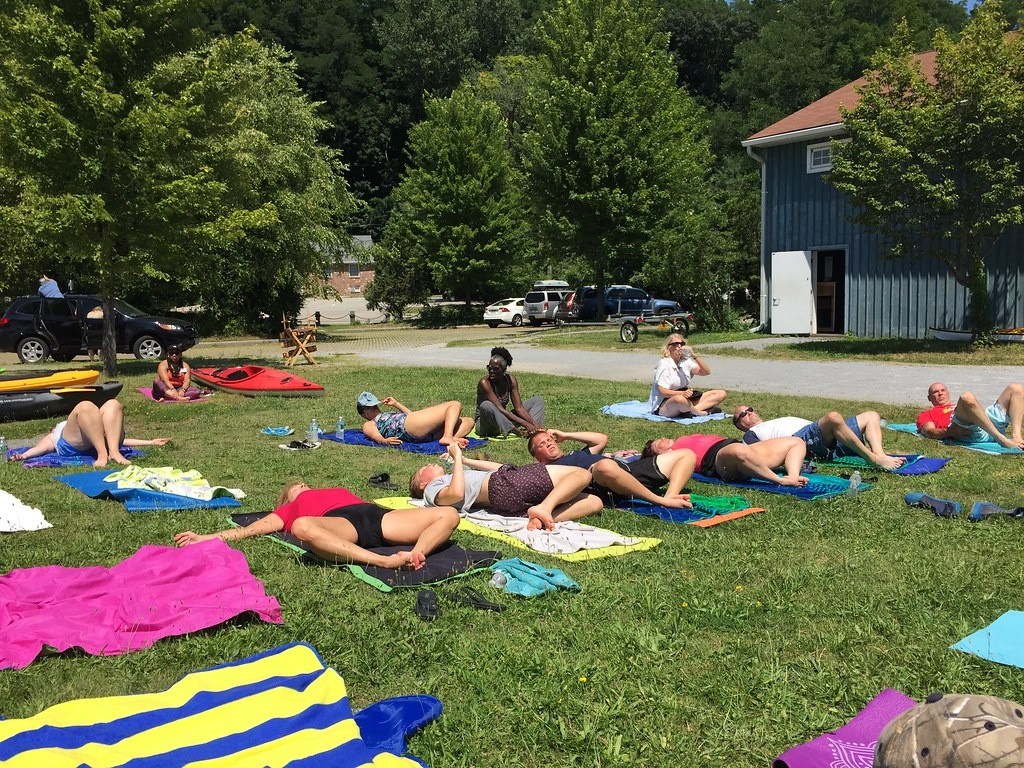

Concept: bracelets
[[693, 355, 698, 360], [379, 437, 384, 444], [179, 388, 186, 392]]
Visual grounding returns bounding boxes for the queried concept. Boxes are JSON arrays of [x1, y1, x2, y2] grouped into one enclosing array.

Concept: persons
[[527, 429, 697, 508], [474, 347, 545, 438], [39, 271, 72, 318], [872, 693, 1024, 768], [916, 383, 1024, 451], [649, 333, 727, 418], [733, 406, 906, 470], [172, 480, 459, 571], [86, 307, 103, 363], [642, 434, 809, 487], [408, 441, 603, 531], [152, 344, 201, 402], [356, 391, 475, 448], [9, 398, 172, 468]]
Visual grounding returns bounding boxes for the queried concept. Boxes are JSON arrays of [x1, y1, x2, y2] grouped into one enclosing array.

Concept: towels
[[101, 464, 248, 502], [598, 400, 735, 426], [884, 423, 1024, 454], [406, 498, 643, 554], [491, 556, 583, 598], [613, 489, 752, 525], [805, 452, 925, 473], [624, 453, 878, 502]]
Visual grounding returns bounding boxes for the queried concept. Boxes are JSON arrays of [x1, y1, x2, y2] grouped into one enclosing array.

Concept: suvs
[[524, 290, 574, 327], [557, 291, 582, 323]]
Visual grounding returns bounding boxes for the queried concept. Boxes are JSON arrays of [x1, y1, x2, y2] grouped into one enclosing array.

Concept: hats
[[166, 345, 181, 352], [870, 690, 1024, 768], [356, 391, 383, 407]]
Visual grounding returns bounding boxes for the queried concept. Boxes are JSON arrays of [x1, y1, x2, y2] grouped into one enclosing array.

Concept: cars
[[0, 294, 199, 365], [483, 298, 531, 328]]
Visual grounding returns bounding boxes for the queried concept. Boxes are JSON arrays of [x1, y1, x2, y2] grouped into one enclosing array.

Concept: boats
[[189, 364, 326, 396], [0, 370, 123, 424], [929, 326, 1024, 344]]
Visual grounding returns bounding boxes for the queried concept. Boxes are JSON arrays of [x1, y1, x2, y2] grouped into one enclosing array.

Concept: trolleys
[[617, 313, 690, 343]]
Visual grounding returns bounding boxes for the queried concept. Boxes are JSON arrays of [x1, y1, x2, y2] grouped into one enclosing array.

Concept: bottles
[[335, 417, 345, 439], [849, 471, 861, 488], [309, 419, 319, 442], [0, 436, 8, 464], [678, 349, 692, 357], [488, 572, 507, 588]]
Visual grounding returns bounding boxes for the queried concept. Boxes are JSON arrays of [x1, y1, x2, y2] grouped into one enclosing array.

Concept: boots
[[904, 493, 962, 519], [966, 502, 1024, 522]]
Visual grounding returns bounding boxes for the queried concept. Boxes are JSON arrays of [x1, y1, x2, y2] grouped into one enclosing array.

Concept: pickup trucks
[[577, 285, 680, 317]]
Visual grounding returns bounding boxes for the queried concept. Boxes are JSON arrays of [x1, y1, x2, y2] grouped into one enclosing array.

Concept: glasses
[[736, 407, 753, 423], [485, 364, 501, 374], [669, 342, 687, 347]]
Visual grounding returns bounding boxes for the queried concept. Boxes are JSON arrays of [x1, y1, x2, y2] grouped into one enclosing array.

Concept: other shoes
[[277, 441, 322, 452], [706, 407, 723, 414]]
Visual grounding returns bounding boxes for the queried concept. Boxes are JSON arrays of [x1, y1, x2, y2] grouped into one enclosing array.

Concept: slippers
[[416, 589, 440, 620], [441, 586, 507, 612], [368, 473, 399, 491]]
